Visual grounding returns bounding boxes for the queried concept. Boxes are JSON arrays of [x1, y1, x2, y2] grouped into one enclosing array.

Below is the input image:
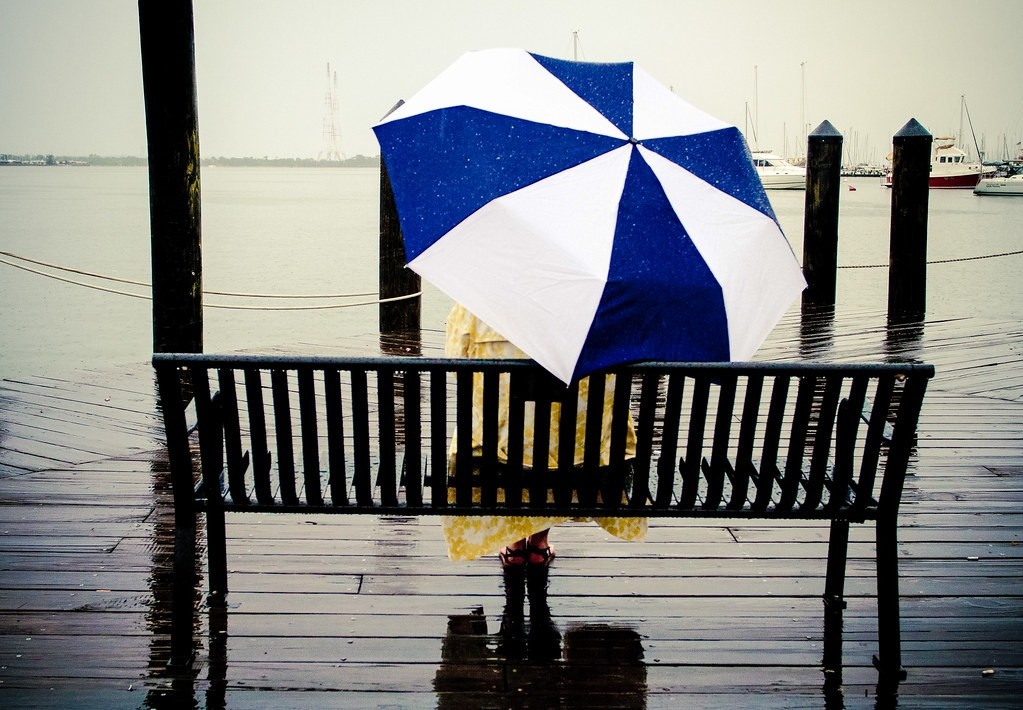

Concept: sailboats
[[842, 129, 888, 176]]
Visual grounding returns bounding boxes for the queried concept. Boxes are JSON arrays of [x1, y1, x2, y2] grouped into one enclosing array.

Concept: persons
[[442, 304, 648, 570]]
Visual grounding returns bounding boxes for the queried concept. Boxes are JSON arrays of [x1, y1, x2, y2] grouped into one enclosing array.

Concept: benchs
[[150, 351, 937, 694]]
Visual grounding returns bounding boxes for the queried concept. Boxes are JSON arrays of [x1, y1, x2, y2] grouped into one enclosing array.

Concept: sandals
[[526, 541, 555, 570], [500, 546, 526, 572]]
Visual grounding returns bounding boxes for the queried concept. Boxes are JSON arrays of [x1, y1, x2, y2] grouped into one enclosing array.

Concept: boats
[[745, 103, 807, 190], [881, 93, 997, 190], [973, 159, 1023, 195]]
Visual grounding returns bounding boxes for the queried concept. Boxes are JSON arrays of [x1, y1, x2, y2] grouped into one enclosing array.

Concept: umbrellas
[[369, 49, 807, 389]]
